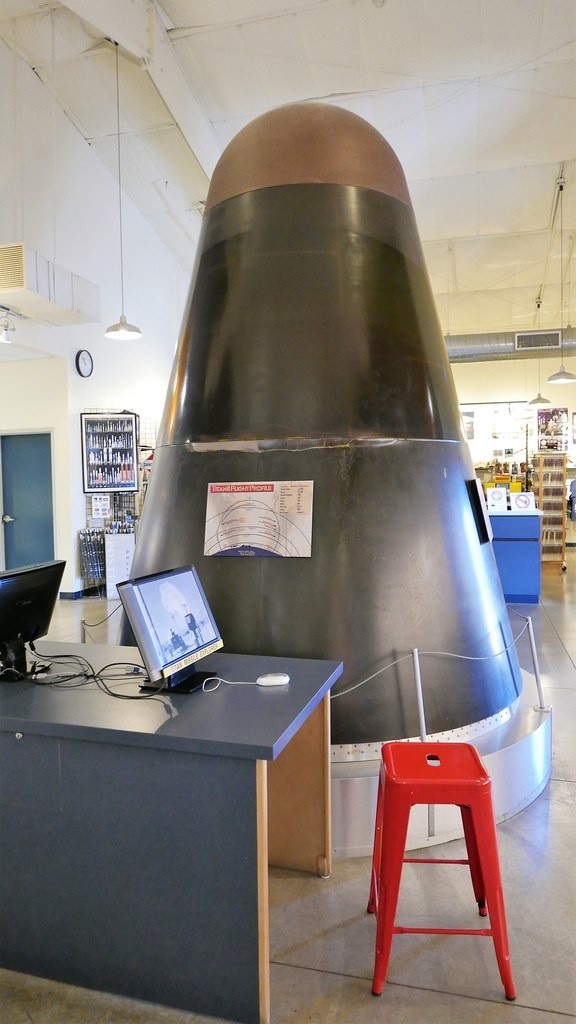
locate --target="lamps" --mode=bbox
[0,321,12,343]
[548,177,576,383]
[105,42,142,341]
[529,299,550,405]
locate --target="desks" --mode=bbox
[0,641,344,1024]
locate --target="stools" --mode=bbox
[366,743,516,1000]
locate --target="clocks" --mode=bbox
[75,350,94,377]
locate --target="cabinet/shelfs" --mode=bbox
[532,452,567,574]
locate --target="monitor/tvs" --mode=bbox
[0,559,66,681]
[116,563,223,693]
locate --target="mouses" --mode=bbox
[256,673,290,687]
